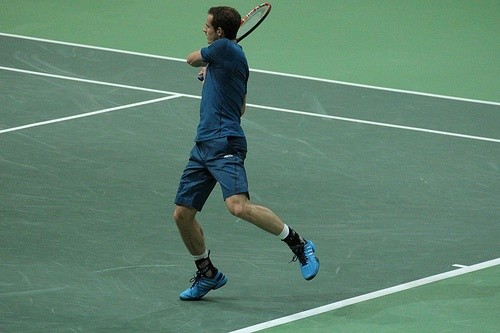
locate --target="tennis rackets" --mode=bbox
[198,1,272,83]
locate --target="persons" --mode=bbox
[172,4,319,301]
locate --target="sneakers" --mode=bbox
[288,238,320,281]
[179,268,227,300]
